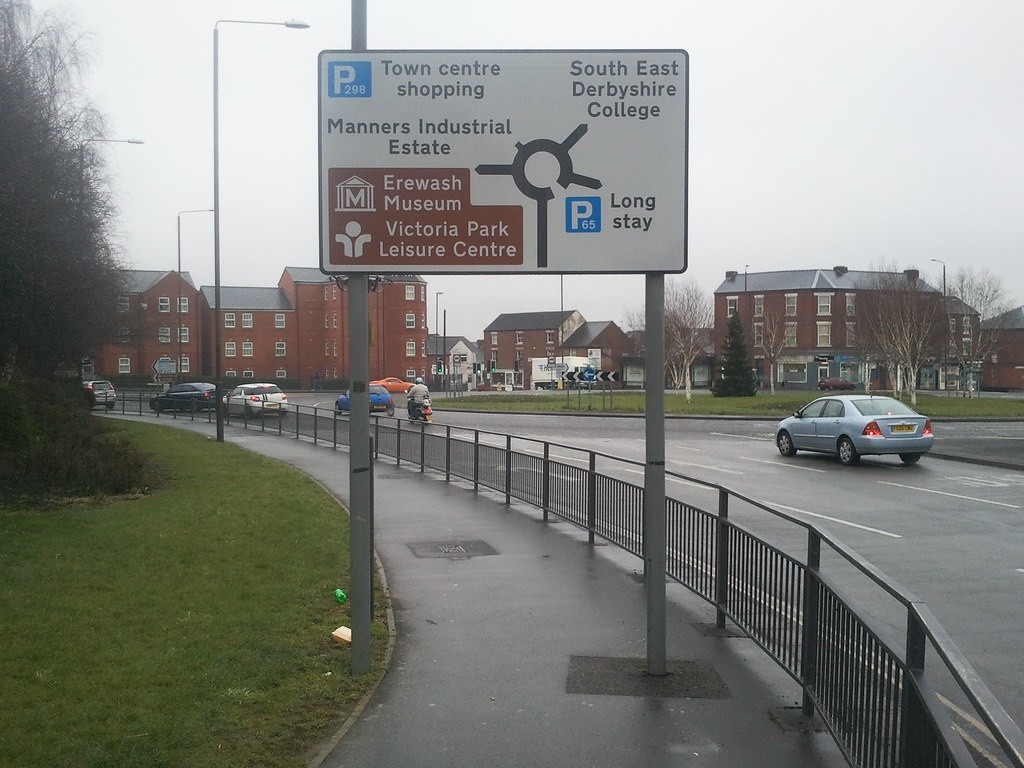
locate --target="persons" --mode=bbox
[407,376,429,419]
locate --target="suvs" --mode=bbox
[82,381,116,409]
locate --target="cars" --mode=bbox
[222,383,288,418]
[368,377,417,393]
[777,395,934,468]
[819,377,856,391]
[150,383,218,413]
[334,384,395,417]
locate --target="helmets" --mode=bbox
[416,377,424,384]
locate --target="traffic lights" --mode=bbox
[477,363,480,373]
[492,361,495,372]
[438,360,442,372]
[514,360,518,371]
[473,363,476,374]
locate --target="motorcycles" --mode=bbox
[405,389,433,424]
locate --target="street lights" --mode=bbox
[77,137,143,198]
[436,292,443,383]
[175,209,216,381]
[930,258,948,390]
[211,16,311,438]
